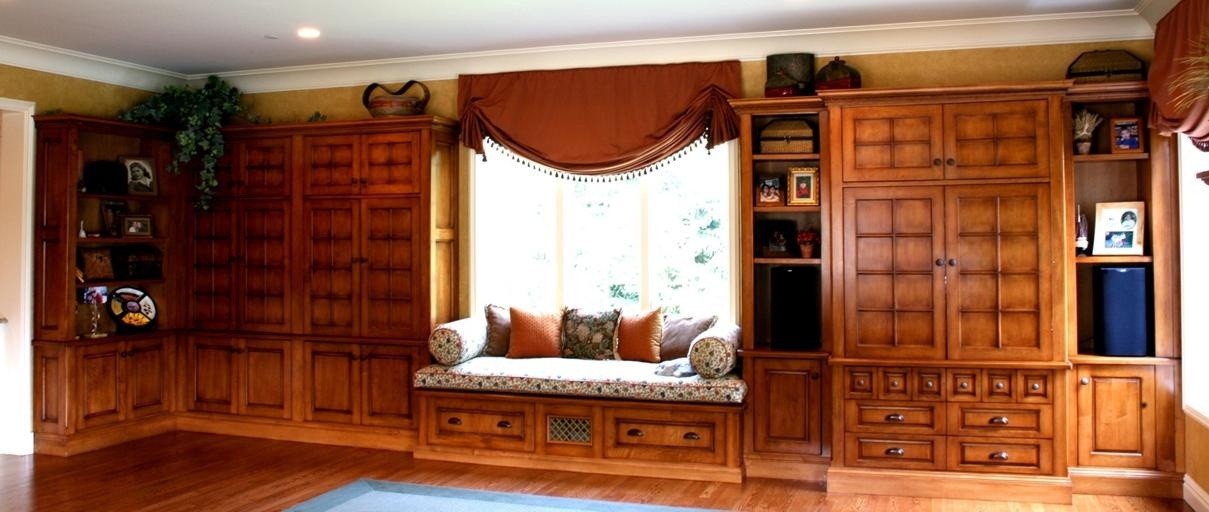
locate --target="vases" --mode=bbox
[1073,140,1094,155]
[799,238,817,259]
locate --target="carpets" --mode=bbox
[265,473,725,512]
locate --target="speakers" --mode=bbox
[1092,267,1145,358]
[772,265,821,352]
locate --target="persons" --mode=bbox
[1120,210,1137,231]
[797,179,809,197]
[768,185,780,202]
[136,221,146,232]
[129,221,138,233]
[1116,127,1139,149]
[125,161,150,191]
[759,184,770,202]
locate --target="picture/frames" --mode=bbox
[1085,197,1148,257]
[1106,113,1146,155]
[117,212,151,237]
[759,218,801,259]
[98,198,131,237]
[784,165,820,207]
[119,152,159,197]
[754,169,788,209]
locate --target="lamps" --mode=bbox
[73,283,113,339]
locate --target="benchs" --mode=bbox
[407,357,753,489]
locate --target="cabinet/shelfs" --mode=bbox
[949,363,1069,479]
[188,191,299,338]
[179,335,301,443]
[722,92,829,356]
[30,107,189,341]
[818,184,1082,369]
[37,341,179,458]
[194,124,303,192]
[300,336,421,455]
[298,197,461,341]
[303,113,460,198]
[601,397,736,472]
[814,71,1068,184]
[741,350,830,467]
[1064,81,1170,361]
[412,390,539,461]
[1069,356,1176,474]
[829,360,948,474]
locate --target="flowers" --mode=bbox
[795,223,820,246]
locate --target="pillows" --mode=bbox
[617,305,665,363]
[502,306,568,359]
[482,302,513,359]
[425,312,488,367]
[559,303,625,362]
[659,311,720,361]
[684,319,743,380]
